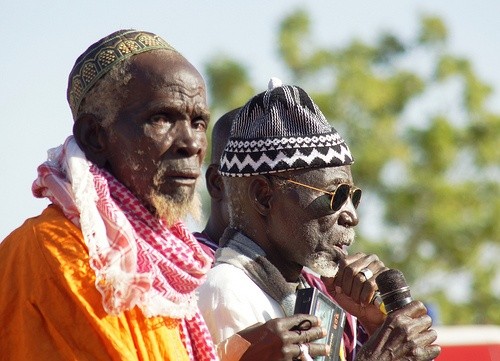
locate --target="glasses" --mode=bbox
[271,174,362,211]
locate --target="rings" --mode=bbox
[298,344,308,360]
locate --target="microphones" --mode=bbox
[377,268,414,314]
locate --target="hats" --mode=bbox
[66,29,176,124]
[217,85,355,178]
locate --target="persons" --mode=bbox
[192,105,242,263]
[0,29,331,361]
[204,87,440,361]
